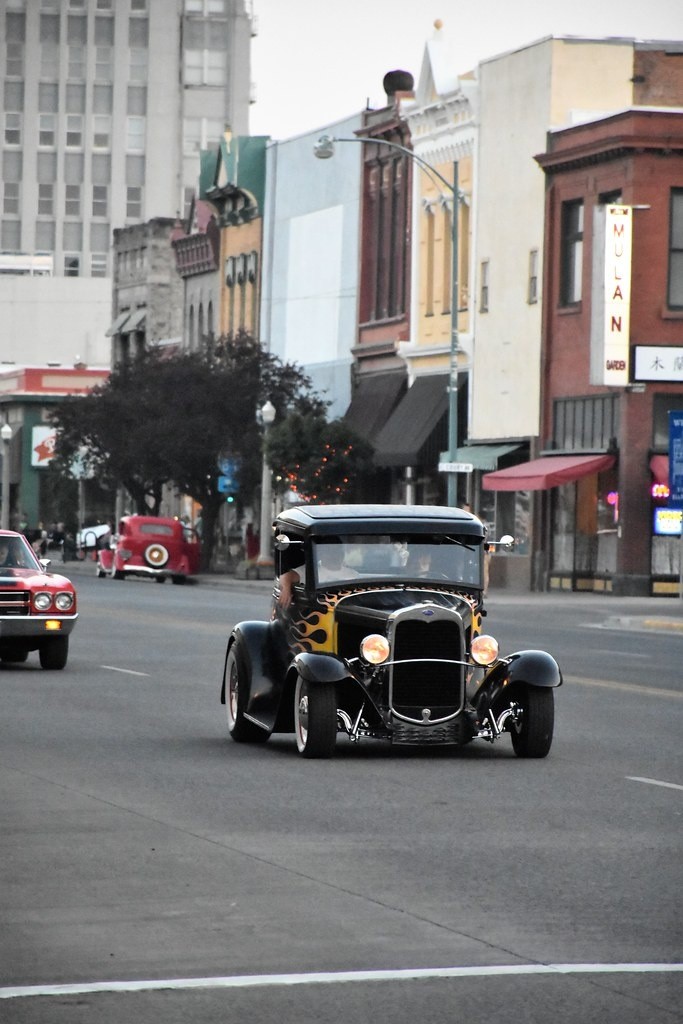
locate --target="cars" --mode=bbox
[97,516,201,585]
[219,504,563,760]
[0,529,78,672]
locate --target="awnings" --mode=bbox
[342,371,616,490]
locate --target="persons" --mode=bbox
[279,534,447,612]
[464,503,492,598]
[0,508,207,570]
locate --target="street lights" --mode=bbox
[313,133,459,507]
[0,422,13,530]
[257,400,277,580]
